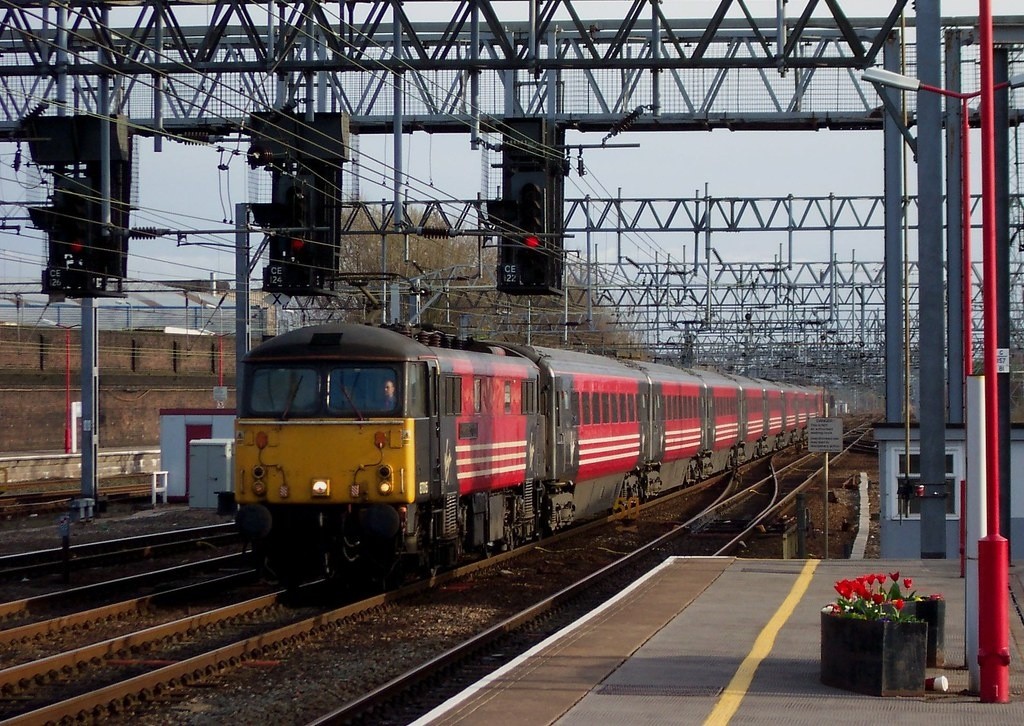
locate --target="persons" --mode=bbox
[372,380,397,410]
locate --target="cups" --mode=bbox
[925,676,948,691]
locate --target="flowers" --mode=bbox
[857,572,942,602]
[822,580,924,622]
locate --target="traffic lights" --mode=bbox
[50,177,98,293]
[274,174,317,269]
[508,173,553,286]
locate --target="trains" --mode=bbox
[227,318,832,606]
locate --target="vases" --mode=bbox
[820,612,929,698]
[890,600,945,668]
